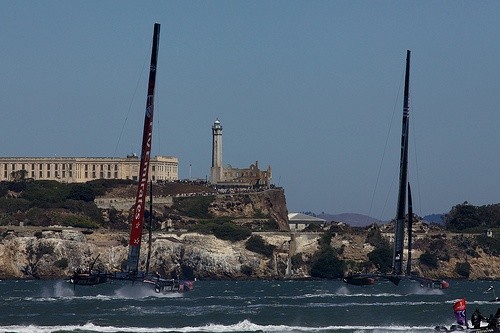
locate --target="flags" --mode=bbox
[452,299,466,327]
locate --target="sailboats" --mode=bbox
[342,45,449,290]
[67,20,195,297]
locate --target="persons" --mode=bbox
[68,266,163,284]
[346,263,397,280]
[471,308,483,329]
